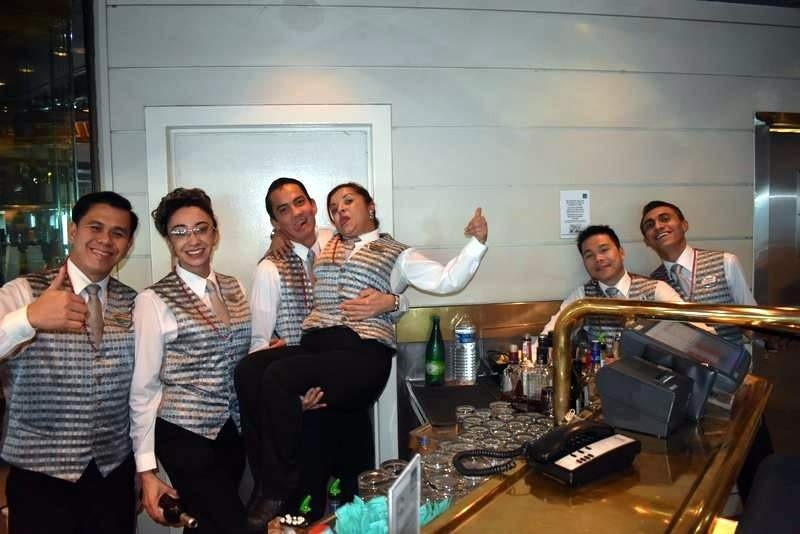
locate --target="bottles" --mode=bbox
[139,488,198,529]
[296,494,312,528]
[425,315,445,385]
[501,330,620,414]
[326,478,342,515]
[0,223,64,287]
[450,309,477,385]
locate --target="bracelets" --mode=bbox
[394,295,400,311]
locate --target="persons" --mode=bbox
[233,178,487,534]
[639,201,758,346]
[0,191,139,534]
[532,226,718,367]
[127,188,252,534]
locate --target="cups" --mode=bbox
[356,386,558,506]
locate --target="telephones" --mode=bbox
[526,420,642,488]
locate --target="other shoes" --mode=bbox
[248,498,285,526]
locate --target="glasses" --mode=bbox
[168,222,217,241]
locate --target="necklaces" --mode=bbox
[298,260,310,315]
[83,321,97,352]
[175,268,228,342]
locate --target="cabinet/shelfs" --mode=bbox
[0,4,79,287]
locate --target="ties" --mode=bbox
[206,279,231,326]
[605,287,619,298]
[306,249,316,285]
[670,264,691,297]
[85,285,104,347]
[343,235,360,260]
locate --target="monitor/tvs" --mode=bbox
[636,322,753,393]
